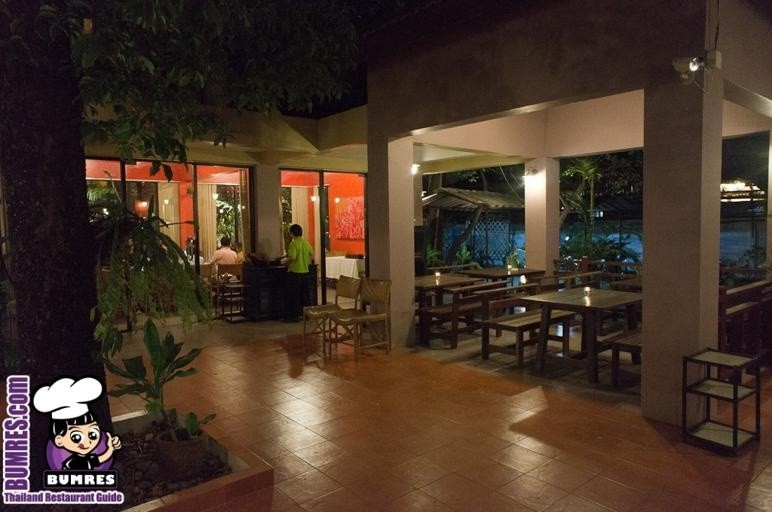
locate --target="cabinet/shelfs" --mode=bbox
[682,347,761,457]
[222,283,252,324]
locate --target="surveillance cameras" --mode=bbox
[671,56,699,73]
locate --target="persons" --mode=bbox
[207,237,236,272]
[280,224,315,320]
[234,242,243,263]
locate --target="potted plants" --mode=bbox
[98,318,216,473]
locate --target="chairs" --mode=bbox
[215,263,245,319]
[303,275,363,360]
[190,263,213,301]
[329,278,392,363]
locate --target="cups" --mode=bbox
[584,286,592,294]
[435,271,441,281]
[506,264,513,272]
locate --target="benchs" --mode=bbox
[415,265,643,387]
